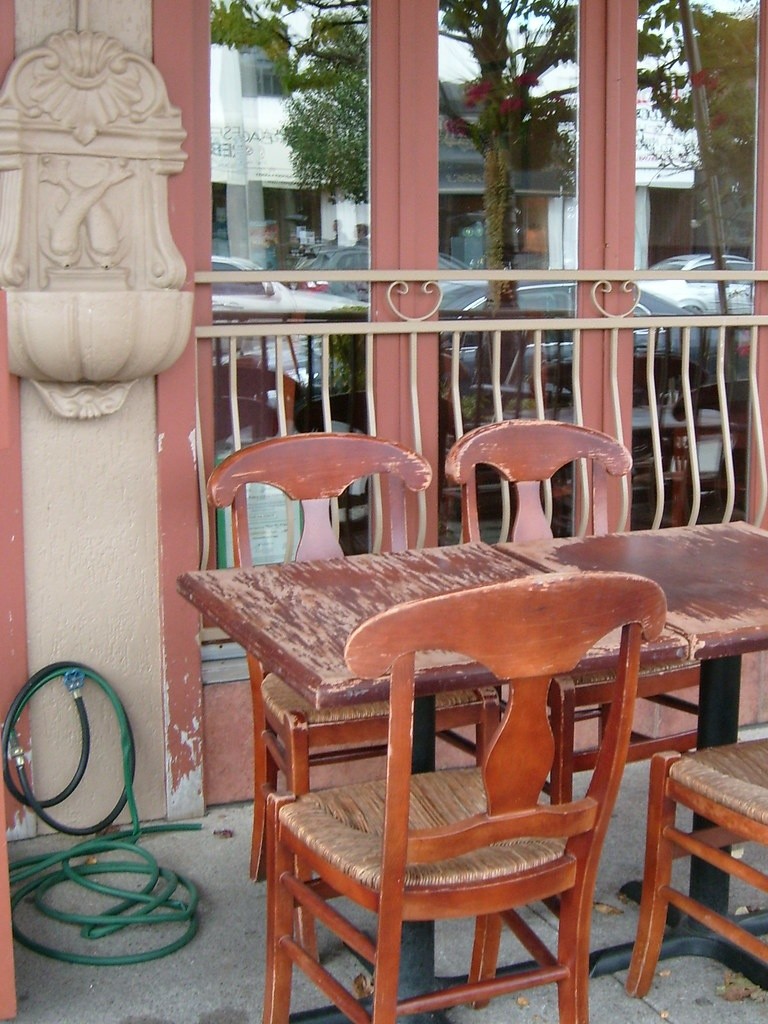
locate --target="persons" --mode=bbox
[327,219,338,245]
[353,223,369,246]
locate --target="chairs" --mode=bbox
[670,380,752,529]
[204,432,497,968]
[625,737,768,1004]
[442,417,704,912]
[263,571,664,1024]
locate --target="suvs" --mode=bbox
[289,245,474,301]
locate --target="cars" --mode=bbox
[211,254,362,354]
[632,254,755,328]
[436,268,754,415]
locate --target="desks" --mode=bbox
[178,540,687,1024]
[490,520,768,1001]
[494,404,736,530]
